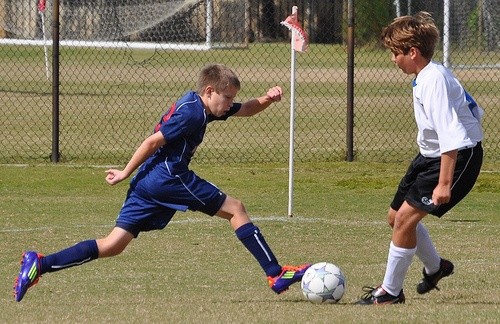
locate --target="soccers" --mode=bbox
[300,262,346,304]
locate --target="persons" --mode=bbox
[355,13,484,305]
[13,63,316,304]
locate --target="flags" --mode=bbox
[281,12,308,54]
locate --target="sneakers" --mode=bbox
[14,250,45,302]
[417,257,454,294]
[354,287,406,306]
[267,264,311,294]
